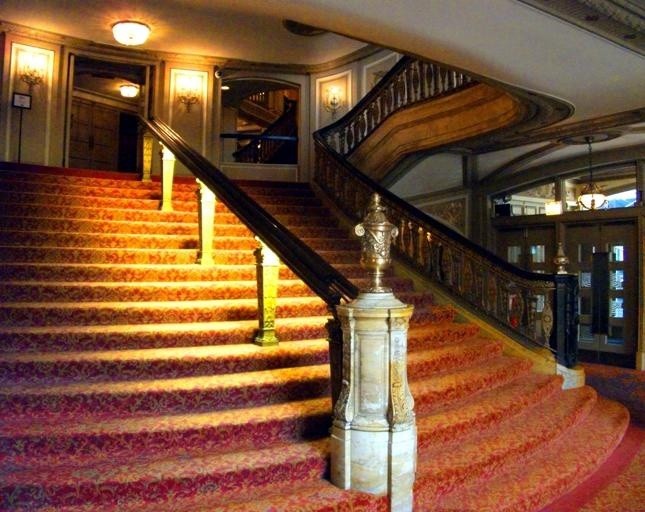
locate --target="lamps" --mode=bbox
[324,87,343,119]
[119,84,140,99]
[577,136,608,212]
[19,58,44,95]
[110,21,151,45]
[177,83,200,113]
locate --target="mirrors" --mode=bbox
[220,77,301,165]
